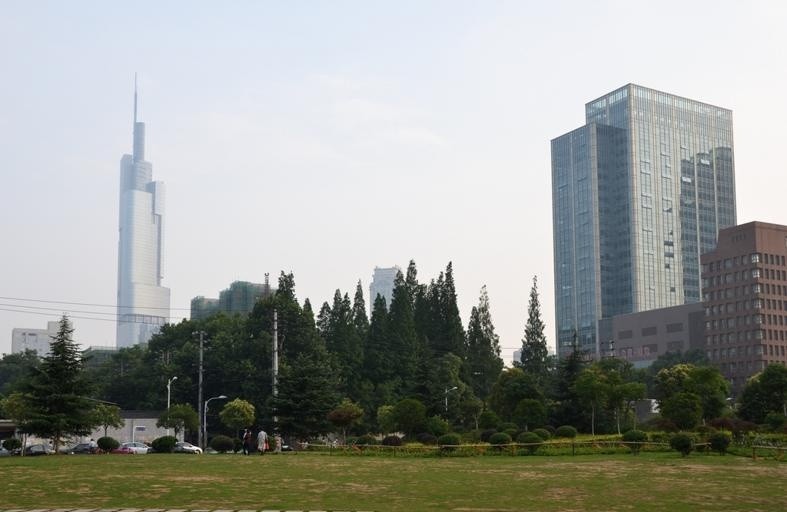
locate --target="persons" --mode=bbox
[273,434,284,454]
[243,429,250,455]
[242,428,251,454]
[47,441,52,455]
[88,438,95,454]
[256,429,268,456]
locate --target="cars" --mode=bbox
[0,441,154,456]
[173,442,202,455]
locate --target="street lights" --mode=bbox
[203,394,227,449]
[444,387,460,424]
[166,376,179,435]
[191,330,207,454]
